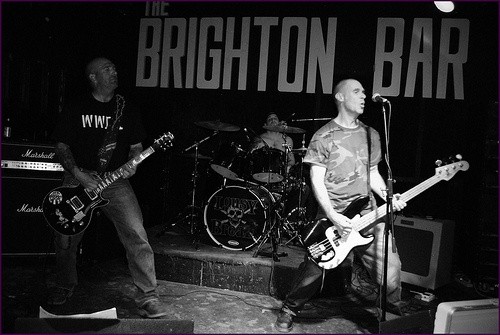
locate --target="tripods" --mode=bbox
[253,129,311,263]
[160,131,218,237]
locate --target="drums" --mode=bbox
[272,176,309,225]
[208,138,251,180]
[200,181,278,252]
[248,146,289,184]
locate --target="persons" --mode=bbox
[274,79,407,333]
[250,112,296,174]
[46,57,167,319]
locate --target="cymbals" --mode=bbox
[200,120,241,132]
[290,146,309,154]
[260,124,308,134]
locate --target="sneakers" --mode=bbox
[376,293,409,315]
[275,304,296,332]
[133,290,168,317]
[45,282,74,304]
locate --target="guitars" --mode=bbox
[296,152,472,272]
[40,132,174,237]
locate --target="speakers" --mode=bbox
[12,317,195,334]
[2,169,82,259]
[387,214,453,289]
[431,297,498,334]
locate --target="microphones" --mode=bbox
[371,93,391,104]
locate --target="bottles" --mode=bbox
[4,116,12,139]
